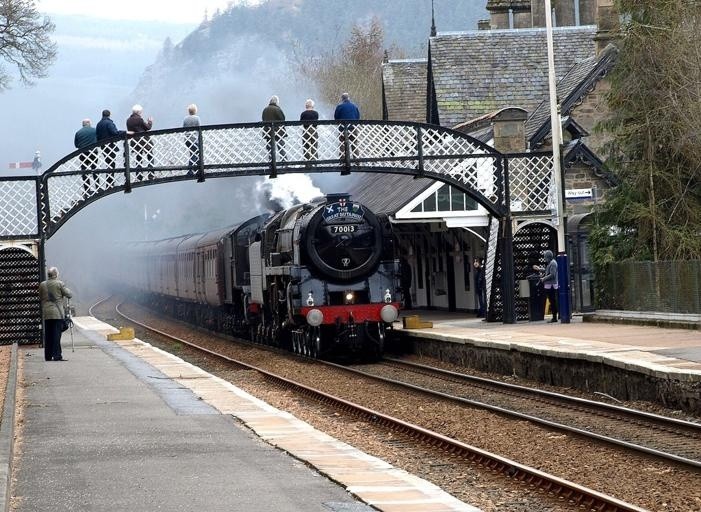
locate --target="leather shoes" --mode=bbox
[61,359,68,361]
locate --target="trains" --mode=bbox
[108,190,405,362]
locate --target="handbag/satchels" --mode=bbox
[61,316,70,331]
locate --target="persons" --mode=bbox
[261,95,290,169]
[182,103,201,175]
[74,120,103,198]
[126,104,154,181]
[39,267,73,361]
[533,249,559,323]
[472,258,481,317]
[396,256,414,310]
[95,109,136,191]
[334,93,361,166]
[300,99,318,168]
[477,260,487,321]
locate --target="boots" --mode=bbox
[547,313,557,323]
